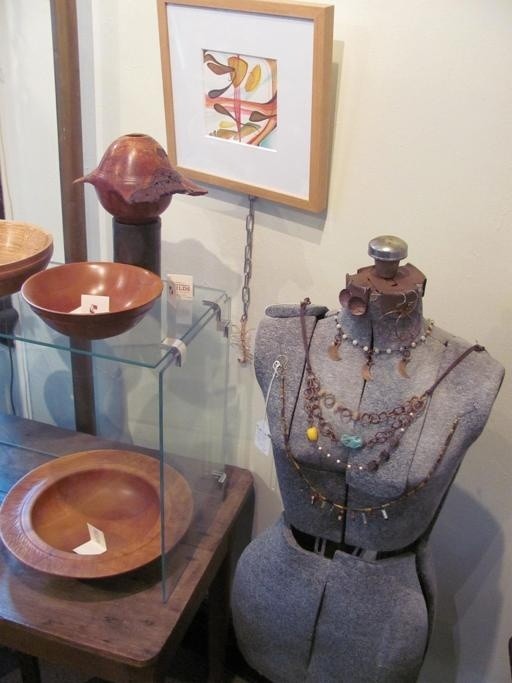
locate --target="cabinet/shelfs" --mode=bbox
[1,258,256,681]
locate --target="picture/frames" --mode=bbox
[157,0,334,214]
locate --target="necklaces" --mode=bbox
[269,298,487,527]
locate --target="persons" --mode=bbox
[230,261,504,681]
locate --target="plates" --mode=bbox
[0,446,196,583]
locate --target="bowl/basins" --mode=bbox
[0,217,56,301]
[19,258,167,341]
